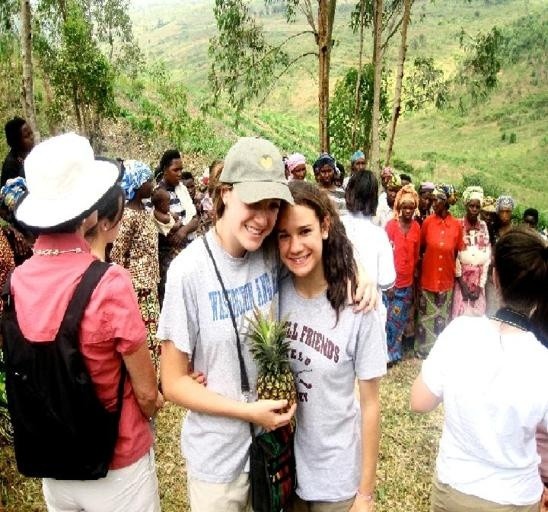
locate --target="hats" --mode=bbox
[13,132,121,232]
[219,135,296,207]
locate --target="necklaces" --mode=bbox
[30,246,85,257]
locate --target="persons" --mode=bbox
[1,132,164,511]
[188,180,391,512]
[409,222,546,512]
[154,134,383,512]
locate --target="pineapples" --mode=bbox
[257,367,297,413]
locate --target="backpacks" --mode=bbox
[0,258,127,481]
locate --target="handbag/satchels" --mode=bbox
[248,423,298,512]
